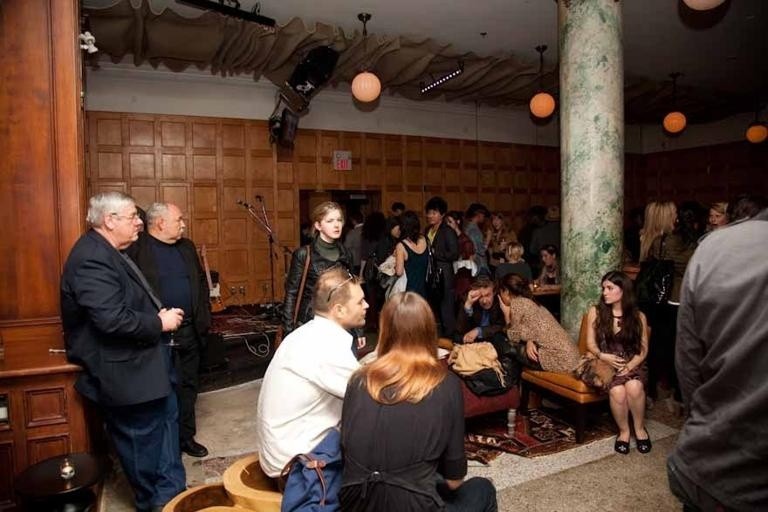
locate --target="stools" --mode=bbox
[160,448,282,511]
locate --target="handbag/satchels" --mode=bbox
[448,341,498,377]
[634,261,674,306]
[281,427,343,512]
[363,251,389,293]
[464,368,512,396]
[426,256,442,288]
[576,351,616,388]
[274,319,302,352]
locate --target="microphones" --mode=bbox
[237,200,255,209]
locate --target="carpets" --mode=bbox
[461,405,625,468]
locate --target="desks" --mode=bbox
[9,450,117,510]
[530,279,559,300]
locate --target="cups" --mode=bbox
[614,351,626,372]
[60,461,74,479]
[534,280,540,288]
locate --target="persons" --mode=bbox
[126,201,212,458]
[59,189,187,512]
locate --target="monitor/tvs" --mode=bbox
[271,108,299,149]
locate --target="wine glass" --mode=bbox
[163,307,181,347]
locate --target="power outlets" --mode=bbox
[262,284,270,292]
[227,285,236,293]
[239,287,244,295]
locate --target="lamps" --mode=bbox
[171,0,277,33]
[417,61,464,95]
[661,72,687,135]
[529,44,556,119]
[352,13,382,104]
[681,0,726,11]
[746,86,767,143]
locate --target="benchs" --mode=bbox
[521,308,651,444]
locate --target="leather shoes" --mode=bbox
[180,438,208,457]
[633,426,650,453]
[615,432,629,454]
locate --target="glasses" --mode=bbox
[322,261,355,302]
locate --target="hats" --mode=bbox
[470,204,490,217]
[545,205,561,221]
